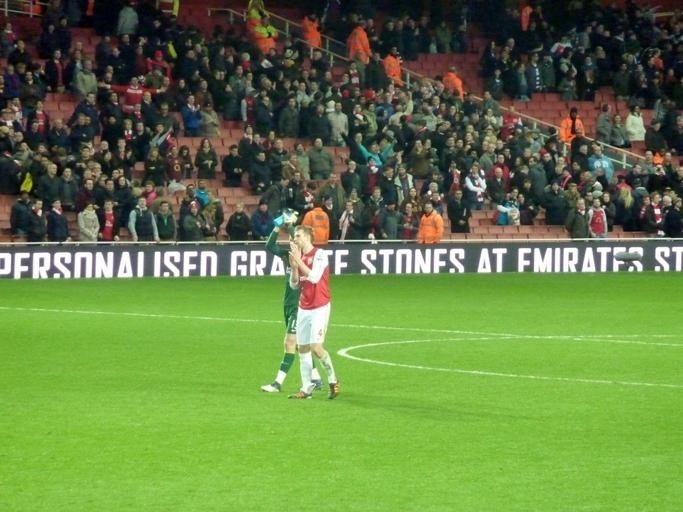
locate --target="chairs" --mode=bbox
[2,4,664,238]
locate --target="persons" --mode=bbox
[286,224,342,400]
[0,0,683,245]
[259,205,325,392]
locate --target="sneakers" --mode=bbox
[286,392,312,400]
[310,378,322,390]
[327,379,340,401]
[260,381,281,393]
